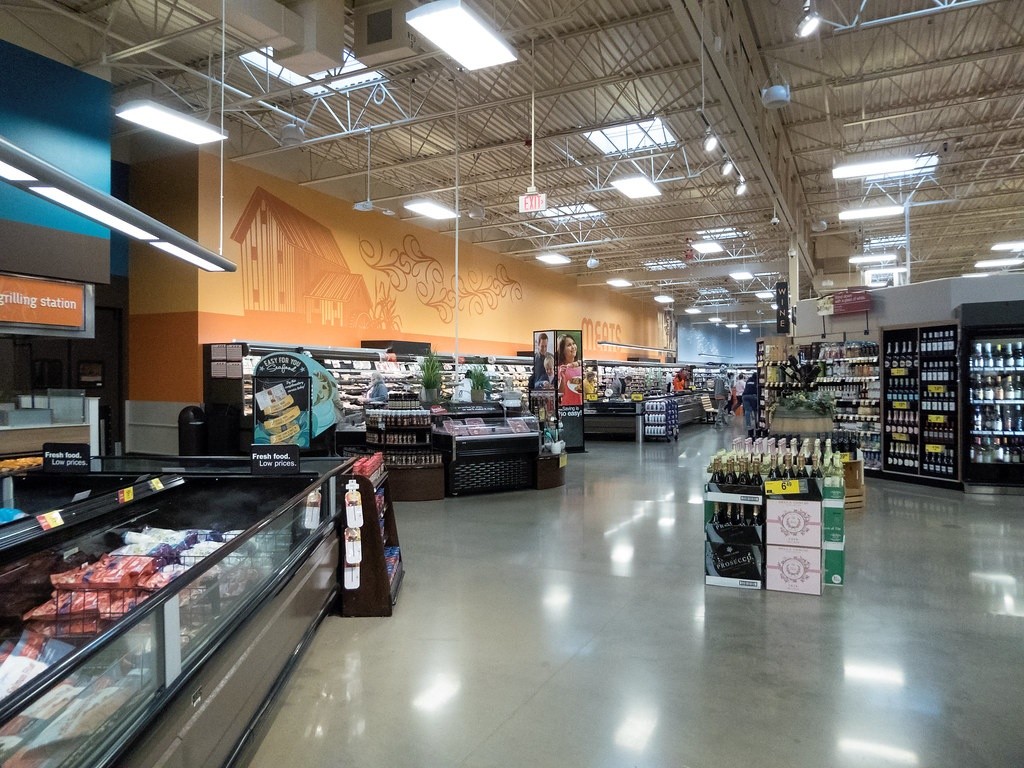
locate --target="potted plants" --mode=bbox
[471,368,492,403]
[417,350,442,403]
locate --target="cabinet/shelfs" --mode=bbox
[754,301,1024,490]
[334,458,405,617]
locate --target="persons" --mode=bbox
[457,370,473,402]
[663,372,673,393]
[343,372,388,422]
[742,372,762,427]
[534,333,554,390]
[557,334,582,406]
[583,372,597,401]
[610,376,632,399]
[713,370,731,429]
[727,372,746,416]
[674,368,691,391]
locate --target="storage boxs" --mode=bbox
[704,493,845,595]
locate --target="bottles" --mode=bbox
[885,329,957,480]
[757,342,767,429]
[345,394,442,464]
[818,341,881,468]
[968,341,1024,466]
[708,436,843,526]
[780,355,820,385]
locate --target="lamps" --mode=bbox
[0,0,1023,332]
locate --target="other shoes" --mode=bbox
[730,410,736,416]
